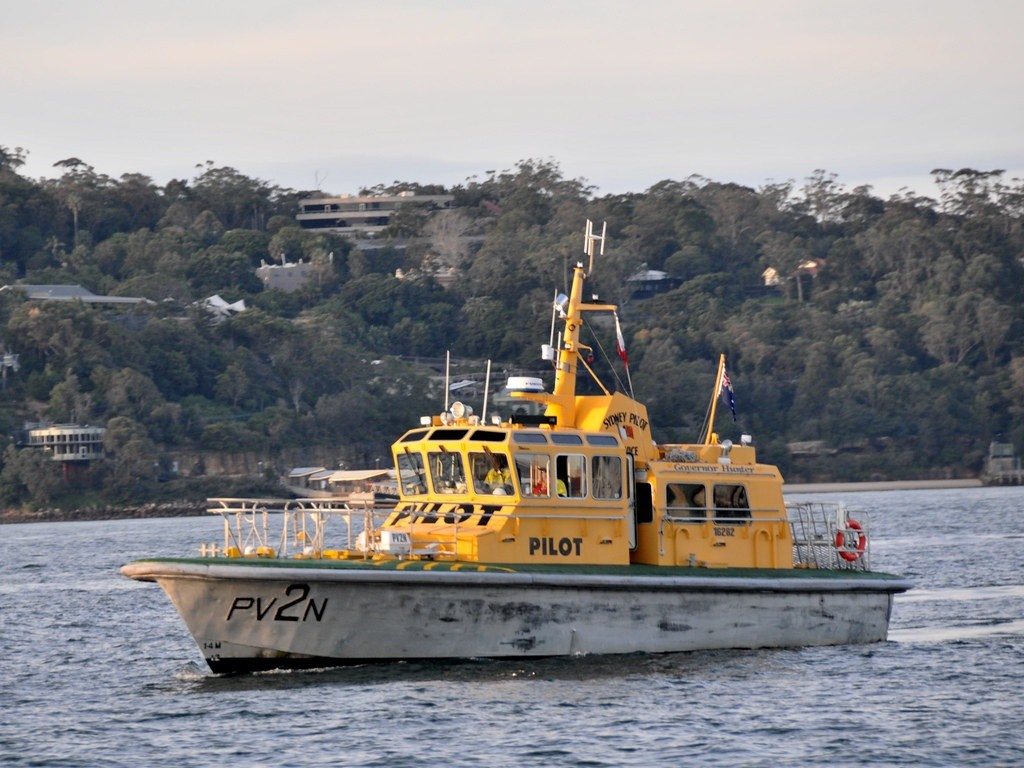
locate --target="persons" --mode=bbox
[533,465,567,498]
[481,452,515,496]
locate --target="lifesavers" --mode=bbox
[833,518,870,563]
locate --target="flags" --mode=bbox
[715,362,737,422]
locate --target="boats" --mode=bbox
[113,223,916,680]
